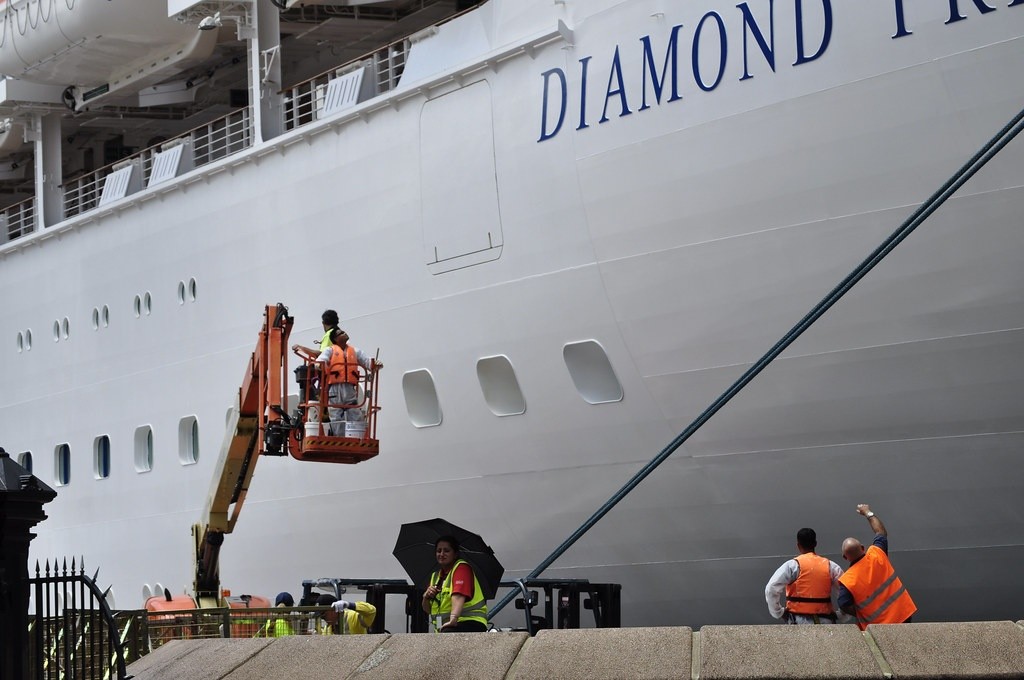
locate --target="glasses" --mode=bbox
[336,331,345,337]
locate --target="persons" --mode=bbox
[836,504,917,626]
[422,538,487,632]
[314,328,382,435]
[765,528,852,623]
[309,594,376,634]
[320,310,339,351]
[265,592,297,637]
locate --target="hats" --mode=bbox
[276,592,294,606]
[312,594,338,619]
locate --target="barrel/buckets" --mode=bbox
[307,401,320,422]
[305,421,330,437]
[345,421,365,439]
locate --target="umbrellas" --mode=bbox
[392,517,504,599]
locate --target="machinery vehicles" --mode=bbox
[143,304,381,646]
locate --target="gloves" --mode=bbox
[331,600,349,613]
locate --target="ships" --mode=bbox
[0,0,1023,680]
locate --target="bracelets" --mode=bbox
[450,614,456,618]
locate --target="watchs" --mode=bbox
[867,512,875,519]
